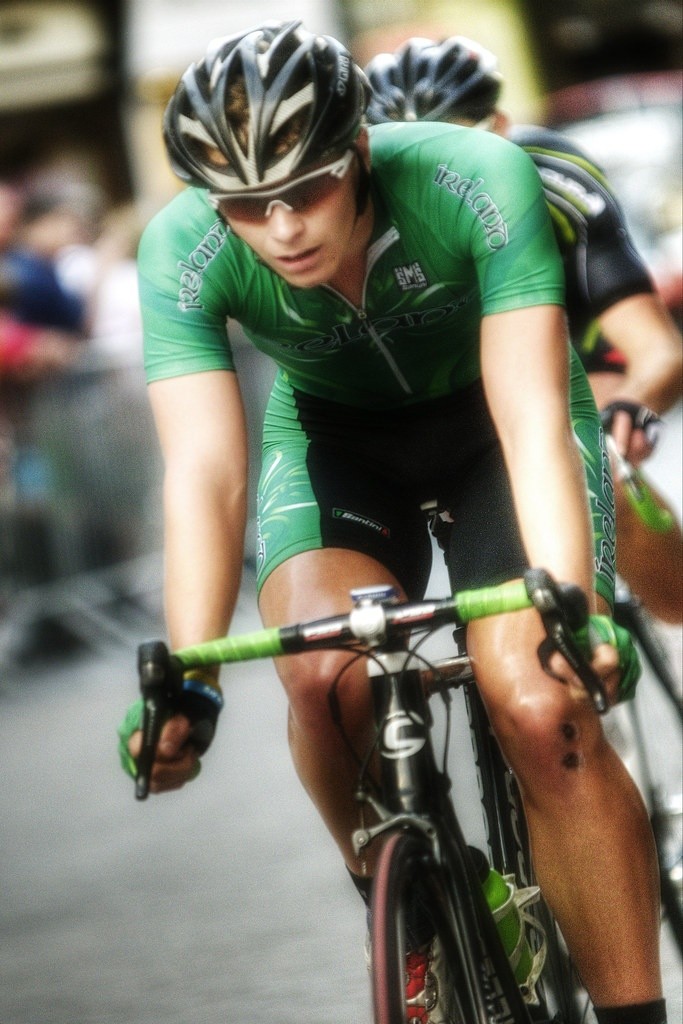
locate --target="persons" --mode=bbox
[119,22,668,1024]
[359,35,683,627]
[0,189,95,376]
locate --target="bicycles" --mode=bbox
[131,497,682,1024]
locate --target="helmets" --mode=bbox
[157,20,372,203]
[368,35,501,125]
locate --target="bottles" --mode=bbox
[466,844,534,985]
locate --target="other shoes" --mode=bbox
[361,929,465,1023]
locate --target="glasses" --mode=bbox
[210,149,356,223]
